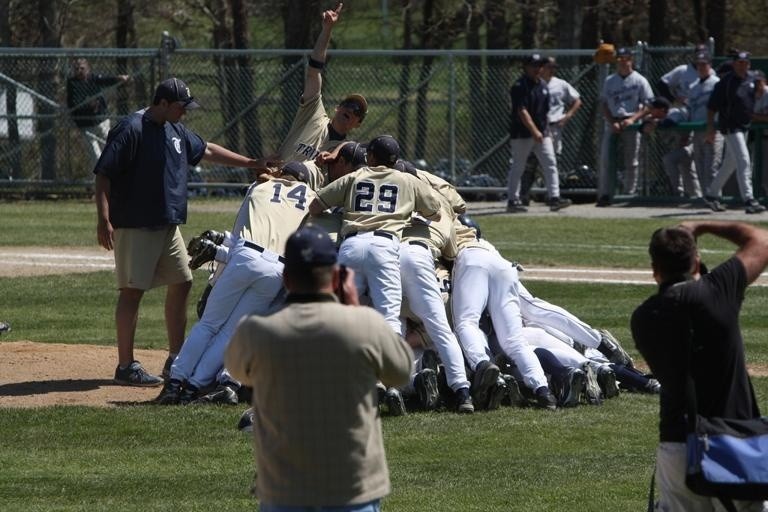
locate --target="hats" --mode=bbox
[616,48,633,57]
[347,93,368,115]
[523,53,560,69]
[646,96,669,113]
[285,227,338,264]
[339,135,416,176]
[458,214,481,239]
[693,45,766,79]
[156,78,201,110]
[282,162,309,184]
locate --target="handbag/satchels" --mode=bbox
[684,414,768,501]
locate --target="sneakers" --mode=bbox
[376,329,662,416]
[154,357,253,405]
[187,230,225,270]
[746,199,766,213]
[115,361,165,387]
[507,195,529,213]
[702,195,727,211]
[547,197,572,211]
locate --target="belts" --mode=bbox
[244,241,285,264]
[409,241,428,249]
[345,232,392,239]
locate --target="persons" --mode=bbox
[92,76,279,388]
[639,46,768,215]
[154,1,660,432]
[224,224,415,512]
[65,59,129,163]
[631,219,768,512]
